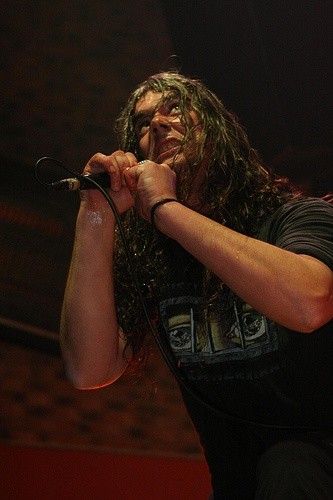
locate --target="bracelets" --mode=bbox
[149,197,180,229]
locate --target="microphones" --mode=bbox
[50,171,111,192]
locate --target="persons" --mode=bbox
[59,71,333,500]
